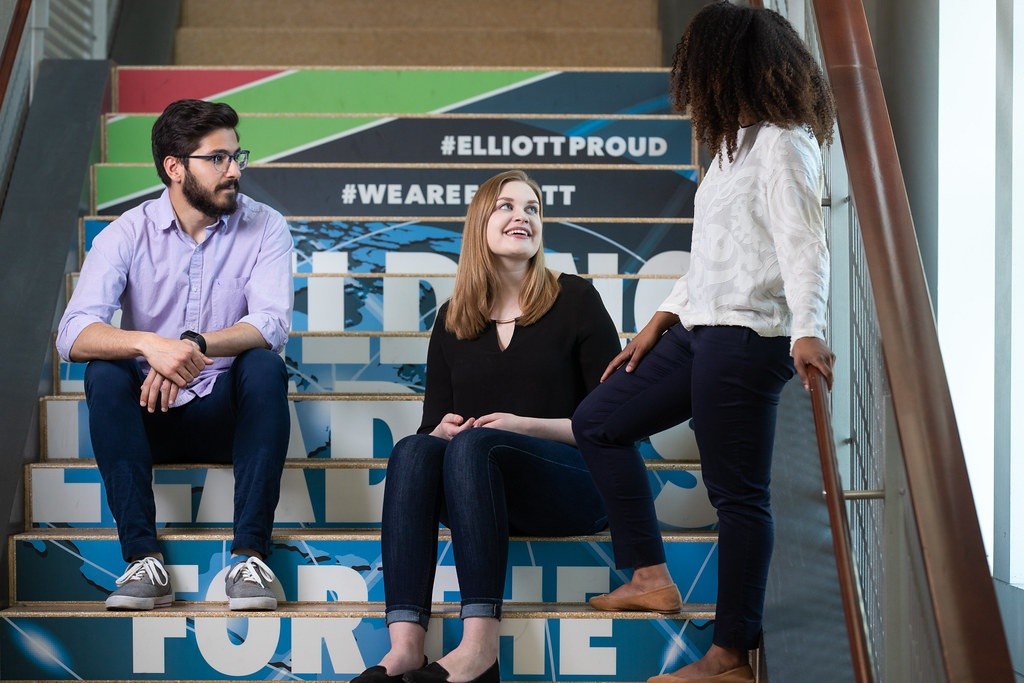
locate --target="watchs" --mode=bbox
[179,329,208,354]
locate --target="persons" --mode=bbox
[350,168,628,683]
[569,7,837,681]
[55,100,294,611]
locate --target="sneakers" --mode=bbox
[225,561,277,611]
[104,558,172,611]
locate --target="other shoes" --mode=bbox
[589,583,683,613]
[648,664,756,683]
[350,655,428,683]
[402,659,502,683]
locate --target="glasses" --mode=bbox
[174,149,250,173]
